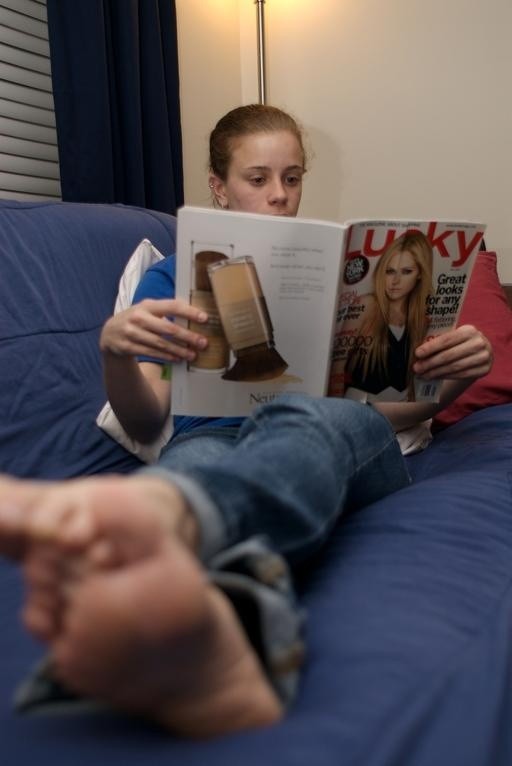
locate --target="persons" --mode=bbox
[331,229,434,402]
[0,104,495,740]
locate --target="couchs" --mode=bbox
[1,199,512,766]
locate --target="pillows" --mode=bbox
[430,249,512,423]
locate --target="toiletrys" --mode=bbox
[204,255,288,381]
[187,251,232,374]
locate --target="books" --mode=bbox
[168,207,488,420]
